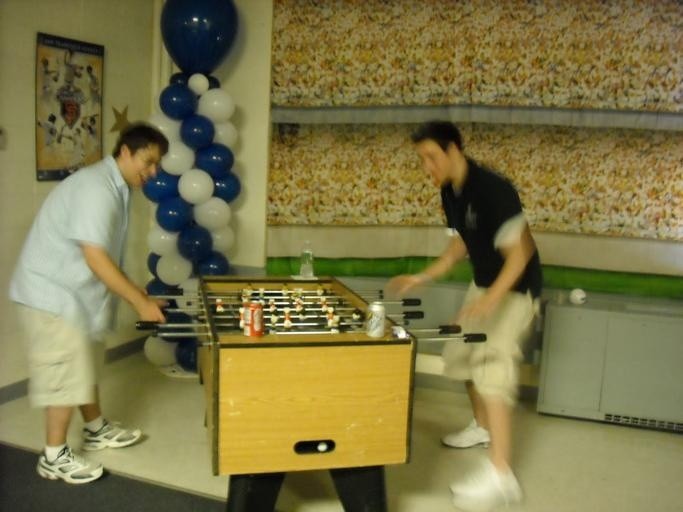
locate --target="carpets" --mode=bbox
[0,434,232,510]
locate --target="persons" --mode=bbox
[6,123,171,487]
[386,121,547,506]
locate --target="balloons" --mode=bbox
[139,68,242,373]
[160,0,237,74]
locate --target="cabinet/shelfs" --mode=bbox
[528,291,682,432]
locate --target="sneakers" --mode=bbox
[79,416,143,453]
[439,416,492,449]
[34,444,108,486]
[447,456,524,512]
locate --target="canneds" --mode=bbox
[244,302,264,337]
[366,301,385,338]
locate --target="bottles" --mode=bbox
[299,237,314,278]
